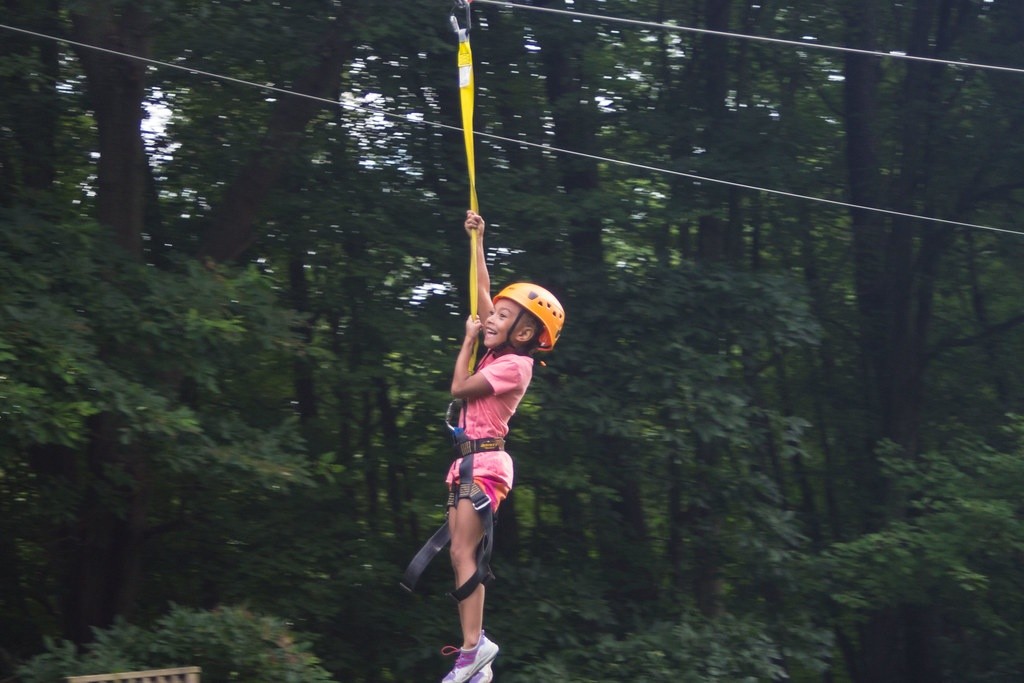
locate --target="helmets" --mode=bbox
[493,283,566,351]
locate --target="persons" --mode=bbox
[439,209,565,683]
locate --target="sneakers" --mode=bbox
[442,630,499,683]
[469,654,495,683]
